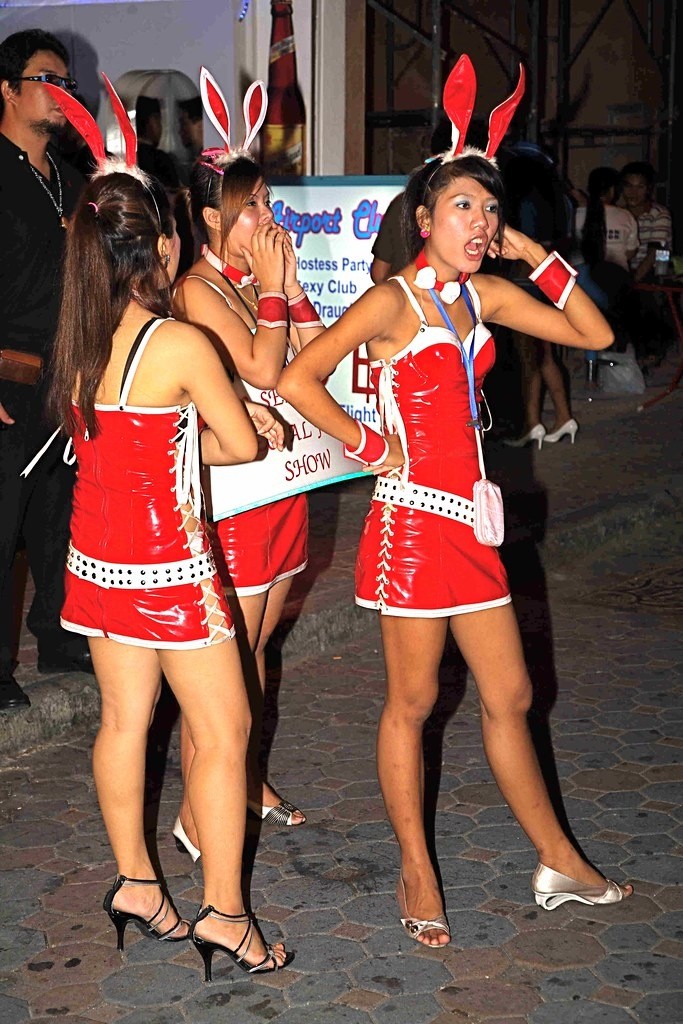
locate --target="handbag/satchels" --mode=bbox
[597,344,645,395]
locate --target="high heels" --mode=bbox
[102,873,193,951]
[187,904,295,982]
[531,862,631,910]
[504,422,546,451]
[543,418,579,445]
[396,868,450,949]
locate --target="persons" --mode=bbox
[275,154,633,948]
[172,157,337,866]
[40,171,284,982]
[136,95,204,186]
[0,29,95,710]
[430,115,671,451]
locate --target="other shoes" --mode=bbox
[247,792,307,827]
[172,815,205,871]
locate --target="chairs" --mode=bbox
[568,262,633,401]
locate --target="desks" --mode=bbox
[632,277,683,412]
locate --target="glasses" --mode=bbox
[17,74,79,92]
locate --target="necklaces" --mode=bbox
[30,152,69,229]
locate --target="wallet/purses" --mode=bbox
[472,478,505,546]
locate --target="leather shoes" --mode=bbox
[36,644,94,676]
[0,677,31,710]
